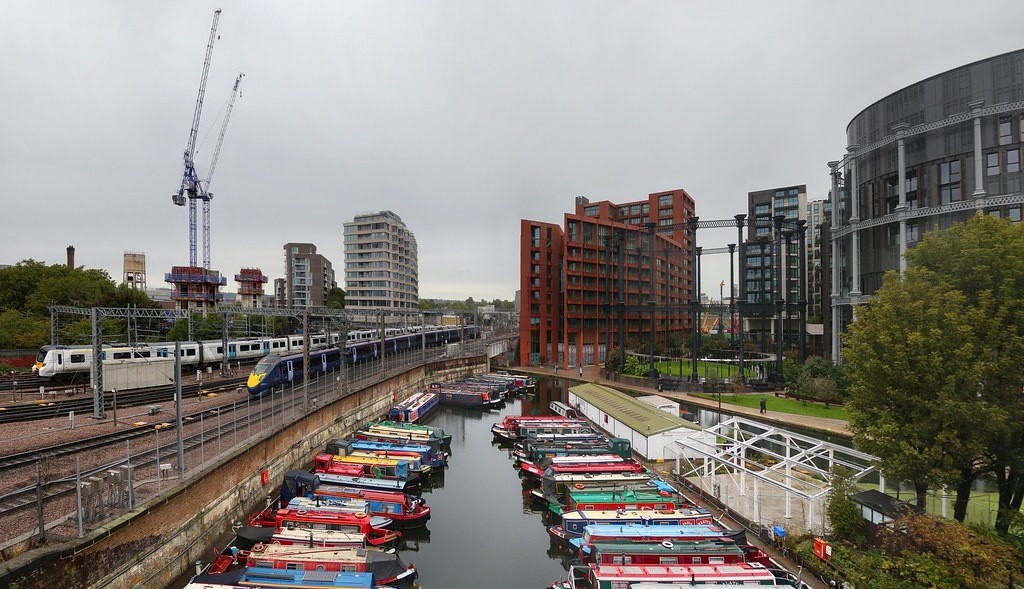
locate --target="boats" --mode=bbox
[492,401,807,589]
[181,422,453,589]
[387,371,538,424]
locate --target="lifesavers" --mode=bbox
[662,541,674,549]
[297,509,308,514]
[562,423,569,427]
[593,439,599,443]
[565,444,572,449]
[253,544,265,552]
[374,424,379,427]
[354,511,367,518]
[658,490,669,496]
[616,508,628,515]
[574,483,585,489]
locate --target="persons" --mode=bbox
[829,580,837,589]
[760,398,767,413]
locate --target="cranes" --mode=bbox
[171,9,247,276]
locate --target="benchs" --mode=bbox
[775,392,789,398]
[798,396,843,404]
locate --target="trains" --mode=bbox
[246,326,481,396]
[34,322,477,383]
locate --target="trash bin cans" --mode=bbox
[658,384,663,392]
[605,372,615,381]
